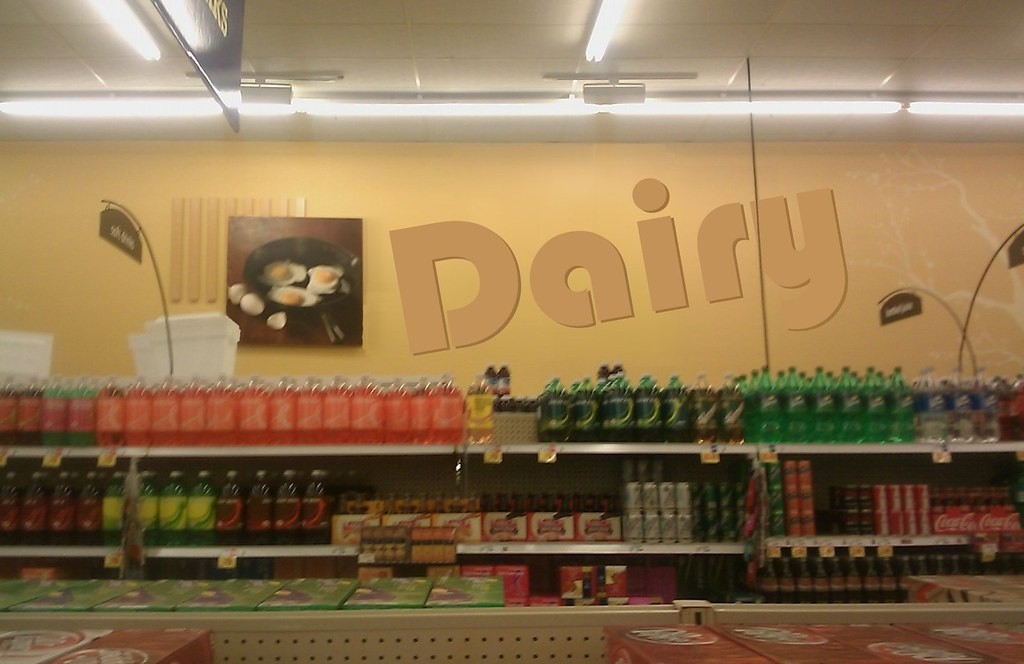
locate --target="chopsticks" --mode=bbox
[321,313,345,344]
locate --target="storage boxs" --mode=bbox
[0,513,678,664]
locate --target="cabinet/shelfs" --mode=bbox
[0,439,1024,581]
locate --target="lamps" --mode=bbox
[0,0,1024,116]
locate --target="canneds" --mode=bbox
[827,483,933,535]
[751,459,814,536]
[621,480,747,544]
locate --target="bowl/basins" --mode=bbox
[244,236,362,313]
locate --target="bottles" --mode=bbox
[898,487,1024,603]
[485,364,497,397]
[98,374,463,447]
[747,556,829,603]
[862,555,897,603]
[480,493,526,513]
[0,375,97,446]
[537,362,717,444]
[386,492,432,513]
[433,491,479,512]
[830,557,861,603]
[335,492,384,514]
[465,371,492,445]
[718,365,914,444]
[359,527,457,564]
[530,492,575,512]
[576,492,620,512]
[497,364,510,397]
[0,470,330,544]
[914,366,1024,444]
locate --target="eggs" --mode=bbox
[306,263,342,295]
[267,285,322,306]
[256,261,307,286]
